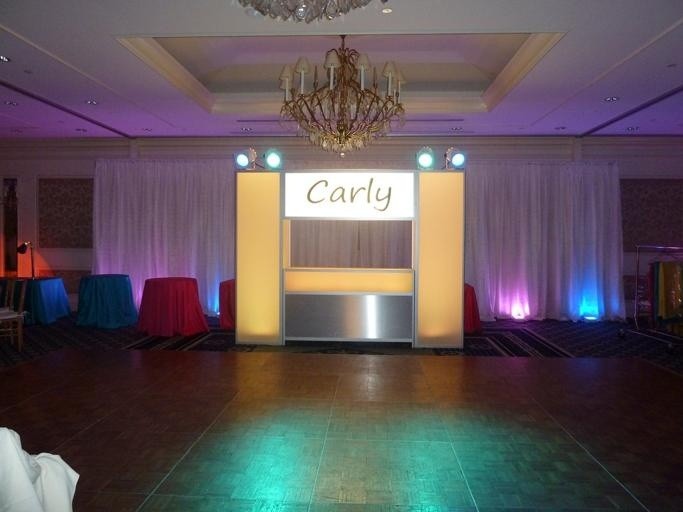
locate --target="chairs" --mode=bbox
[0,277,26,353]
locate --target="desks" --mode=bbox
[24,273,236,338]
[463,282,482,336]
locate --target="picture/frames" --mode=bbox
[36,176,94,251]
[619,176,683,252]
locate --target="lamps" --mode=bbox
[16,241,36,281]
[236,1,393,25]
[235,147,283,171]
[276,34,408,158]
[415,145,467,170]
